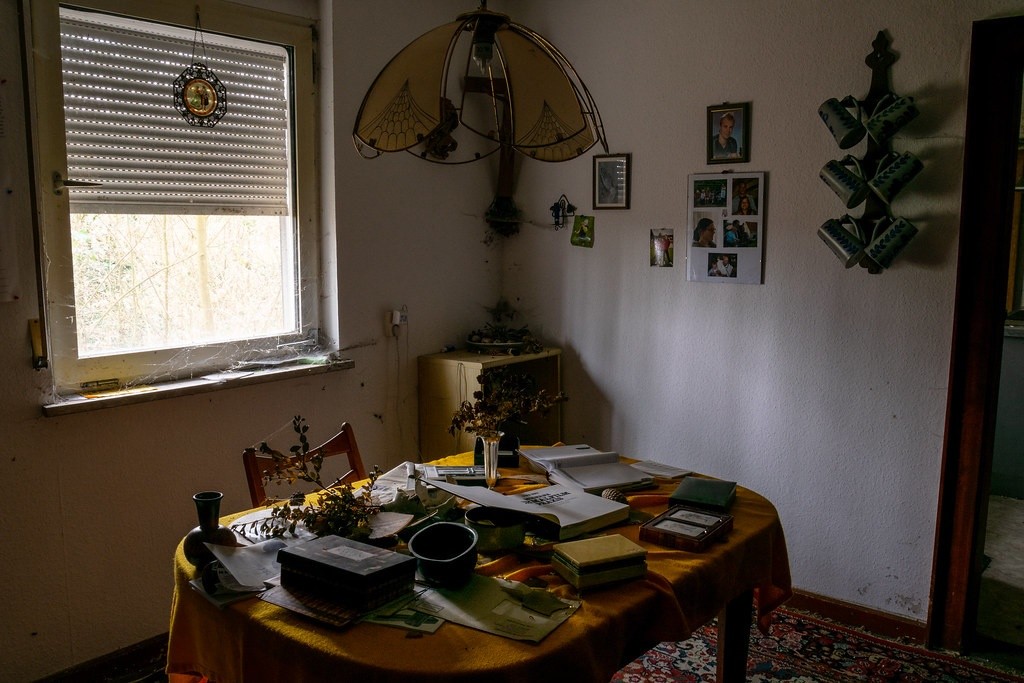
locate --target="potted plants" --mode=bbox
[445,363,570,490]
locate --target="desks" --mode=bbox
[173,444,777,683]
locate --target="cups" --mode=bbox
[408,521,478,587]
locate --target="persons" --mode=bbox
[733,197,758,215]
[695,184,726,205]
[708,256,734,277]
[713,112,737,157]
[576,218,589,238]
[692,218,717,248]
[661,234,672,264]
[726,220,753,247]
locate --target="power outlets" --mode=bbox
[383,310,404,338]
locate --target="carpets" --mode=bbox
[606,601,1024,683]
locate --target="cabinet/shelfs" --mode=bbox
[417,347,562,464]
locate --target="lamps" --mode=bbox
[350,0,610,165]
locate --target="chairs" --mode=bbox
[242,420,368,509]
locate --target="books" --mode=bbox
[516,444,655,495]
[418,477,629,539]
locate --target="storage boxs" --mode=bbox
[639,504,735,554]
[668,476,737,515]
[277,533,417,613]
[551,534,648,599]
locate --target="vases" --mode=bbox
[183,490,238,577]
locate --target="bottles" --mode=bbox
[184,491,236,572]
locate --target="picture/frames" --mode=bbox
[707,102,750,164]
[594,153,631,213]
